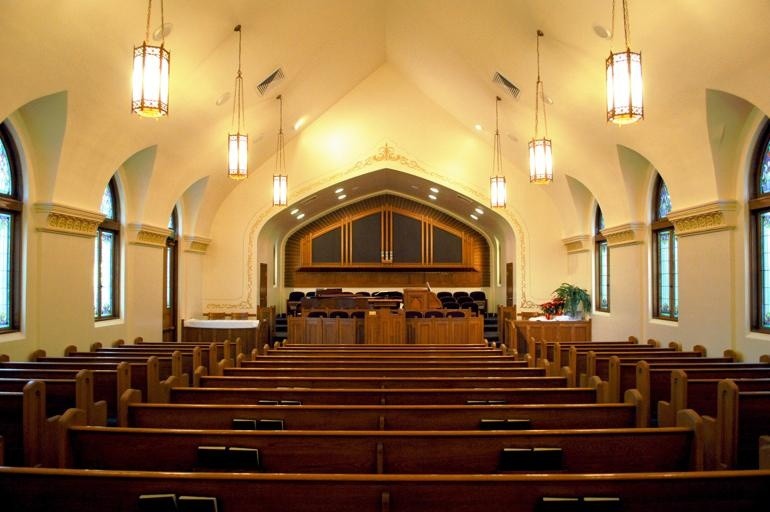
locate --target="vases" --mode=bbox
[544,310,565,321]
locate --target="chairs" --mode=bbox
[283,288,489,324]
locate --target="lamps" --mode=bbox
[489,99,507,209]
[128,1,171,116]
[526,32,555,184]
[225,28,248,178]
[603,1,645,125]
[273,99,287,206]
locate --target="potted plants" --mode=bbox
[556,281,592,318]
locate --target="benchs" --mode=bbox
[0,334,547,335]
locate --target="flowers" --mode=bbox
[541,298,565,313]
